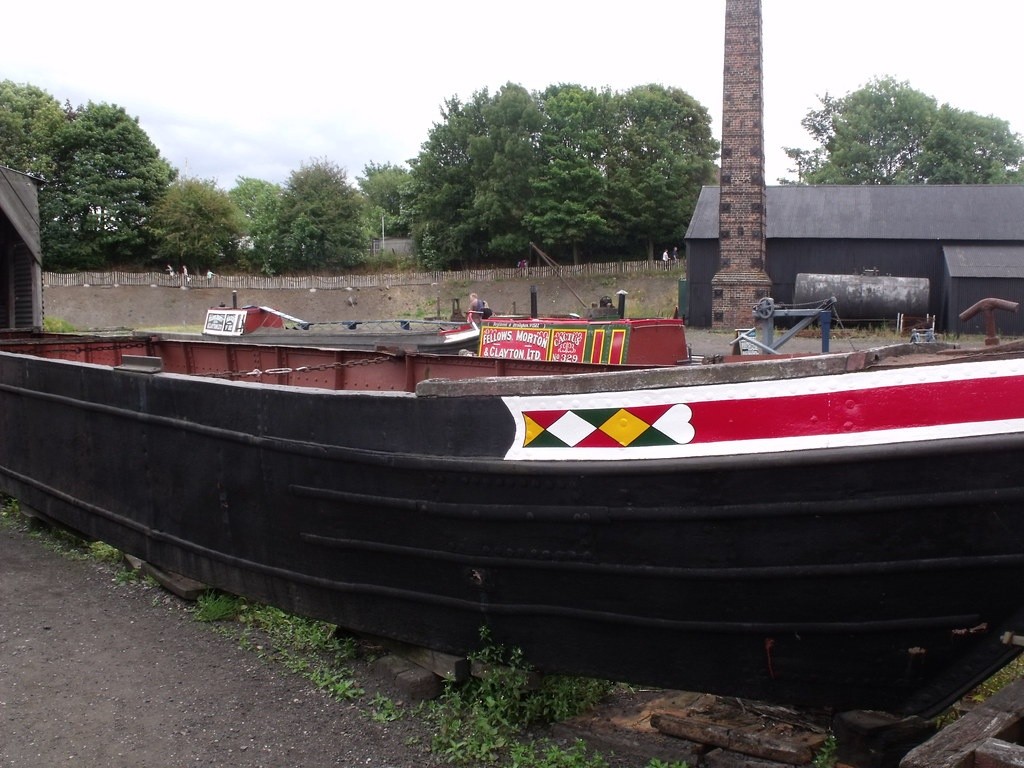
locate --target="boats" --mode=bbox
[10,312,1023,722]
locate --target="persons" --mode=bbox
[662,247,680,270]
[469,293,488,311]
[521,260,527,268]
